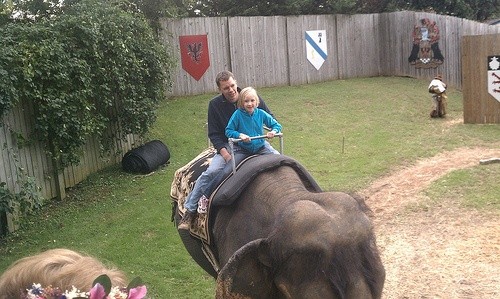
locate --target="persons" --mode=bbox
[177,70,277,231]
[197,87,283,214]
[0,249,147,299]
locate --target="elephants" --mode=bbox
[171,146,386,299]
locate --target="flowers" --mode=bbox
[23,274,148,299]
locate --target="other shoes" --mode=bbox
[197,195,209,214]
[177,210,196,230]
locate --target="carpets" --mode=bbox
[171,144,261,245]
